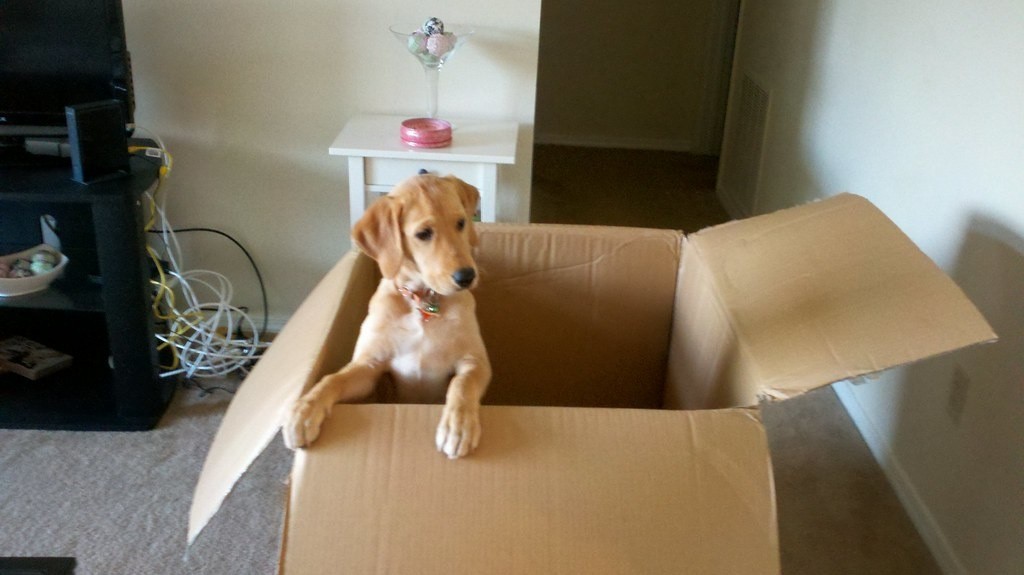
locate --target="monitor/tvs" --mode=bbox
[0,0,135,143]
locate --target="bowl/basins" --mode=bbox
[0,243,70,299]
[400,118,453,149]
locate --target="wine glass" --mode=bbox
[391,23,475,119]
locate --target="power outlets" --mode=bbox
[947,365,969,428]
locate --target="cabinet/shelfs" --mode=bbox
[0,153,177,432]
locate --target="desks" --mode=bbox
[328,113,519,249]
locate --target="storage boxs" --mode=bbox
[186,190,1000,575]
[0,334,74,380]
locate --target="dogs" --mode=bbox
[283,173,491,461]
